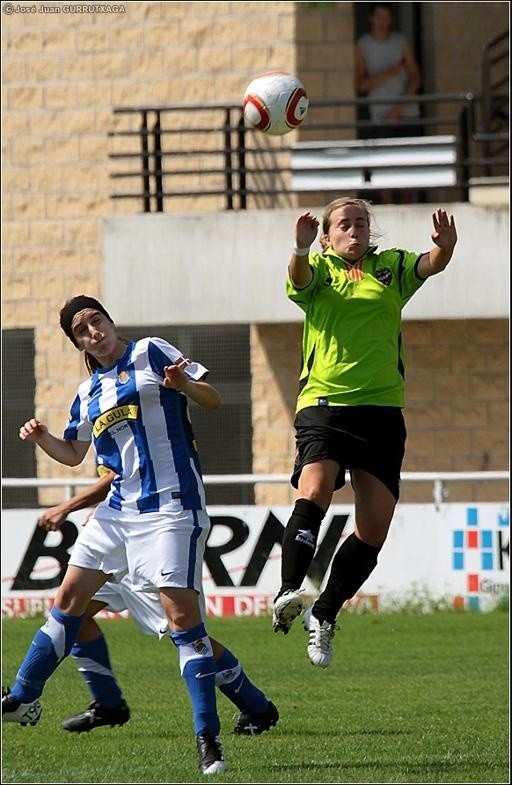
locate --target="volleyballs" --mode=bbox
[244,71,308,135]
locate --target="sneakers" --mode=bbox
[301,605,335,669]
[232,701,279,737]
[271,588,304,635]
[1,694,41,728]
[62,699,129,734]
[195,733,226,775]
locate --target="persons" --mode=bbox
[355,4,424,204]
[272,195,456,668]
[36,468,279,737]
[2,294,224,775]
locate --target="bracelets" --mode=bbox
[291,243,310,257]
[176,374,189,391]
